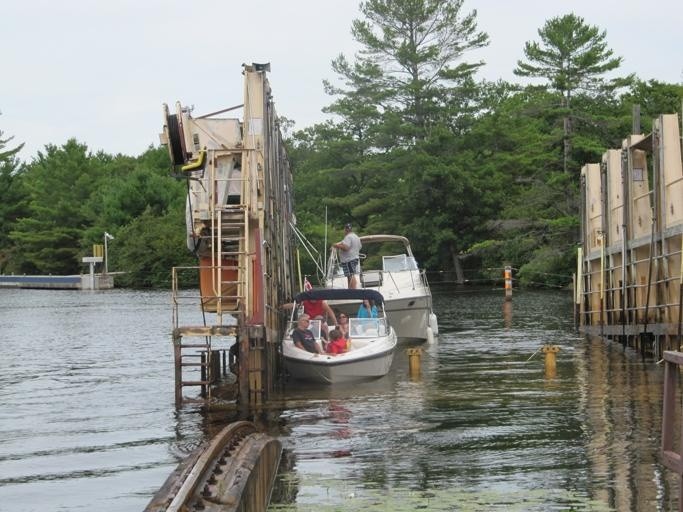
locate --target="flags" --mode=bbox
[304,279,312,291]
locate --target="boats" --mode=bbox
[277,285,401,387]
[323,230,442,349]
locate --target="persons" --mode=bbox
[310,316,329,349]
[338,331,350,352]
[332,222,362,289]
[292,315,321,353]
[279,296,338,326]
[336,312,349,338]
[325,330,346,354]
[357,299,377,318]
